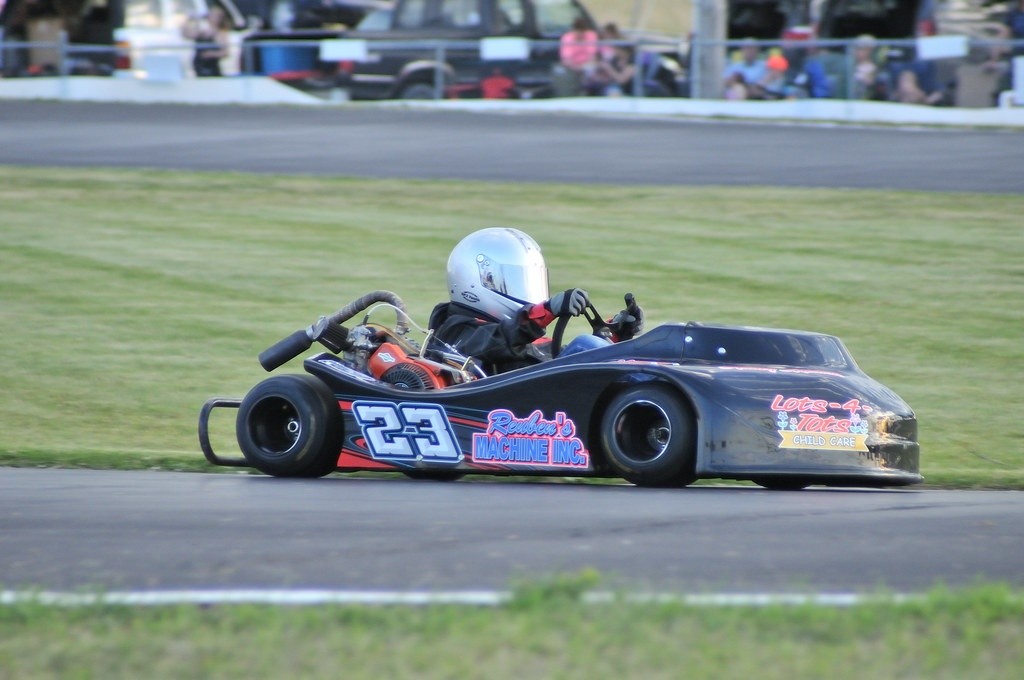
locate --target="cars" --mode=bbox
[60,0,253,78]
[318,0,679,98]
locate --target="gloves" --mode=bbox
[548,288,591,318]
[613,304,644,337]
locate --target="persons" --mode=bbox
[181,4,232,78]
[557,8,1024,110]
[422,227,645,380]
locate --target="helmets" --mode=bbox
[446,227,549,322]
